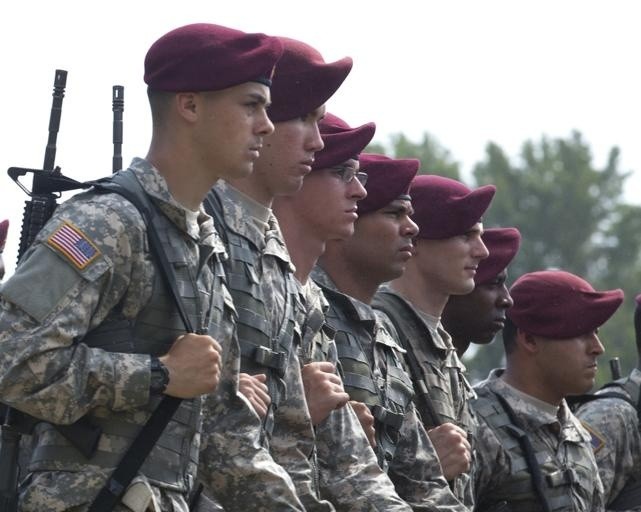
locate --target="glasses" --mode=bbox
[324,167,368,187]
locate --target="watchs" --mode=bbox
[150,356,170,398]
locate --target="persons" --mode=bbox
[473,271,625,512]
[443,226,521,512]
[375,175,495,512]
[578,296,641,512]
[224,36,335,512]
[319,154,468,512]
[274,114,410,512]
[1,23,304,512]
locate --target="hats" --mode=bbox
[506,271,625,340]
[356,153,420,214]
[410,174,496,239]
[474,227,521,285]
[312,111,376,170]
[266,36,353,122]
[144,24,284,93]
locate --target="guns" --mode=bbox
[1,70,101,511]
[113,86,122,175]
[609,357,621,380]
